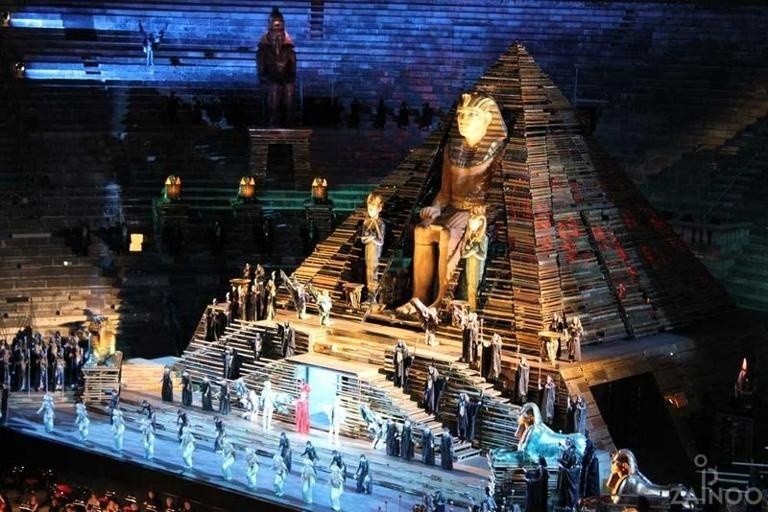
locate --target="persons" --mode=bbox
[395,90,508,329]
[0,262,586,511]
[255,5,297,129]
[459,205,487,312]
[359,193,385,305]
[142,32,155,66]
